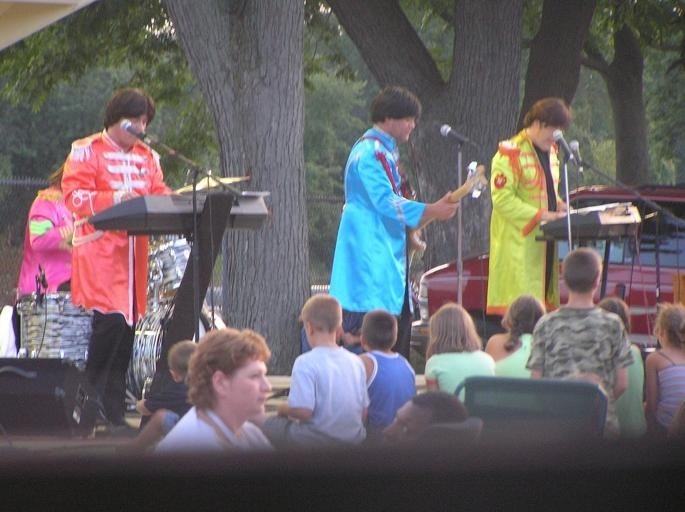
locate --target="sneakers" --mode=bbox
[100,405,129,429]
[72,407,96,439]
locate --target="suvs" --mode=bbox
[417,184,685,354]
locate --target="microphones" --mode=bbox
[568,138,583,165]
[440,124,475,150]
[36,263,50,289]
[119,118,156,152]
[551,129,575,161]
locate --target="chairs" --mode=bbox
[451,373,610,443]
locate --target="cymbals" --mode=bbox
[174,176,251,194]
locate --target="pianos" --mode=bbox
[538,201,642,240]
[89,192,268,235]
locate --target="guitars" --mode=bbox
[406,161,487,268]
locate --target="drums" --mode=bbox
[146,236,192,297]
[125,301,228,411]
[15,292,95,372]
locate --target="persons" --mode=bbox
[327,82,461,360]
[114,245,685,454]
[485,94,573,320]
[60,86,175,439]
[11,164,75,360]
